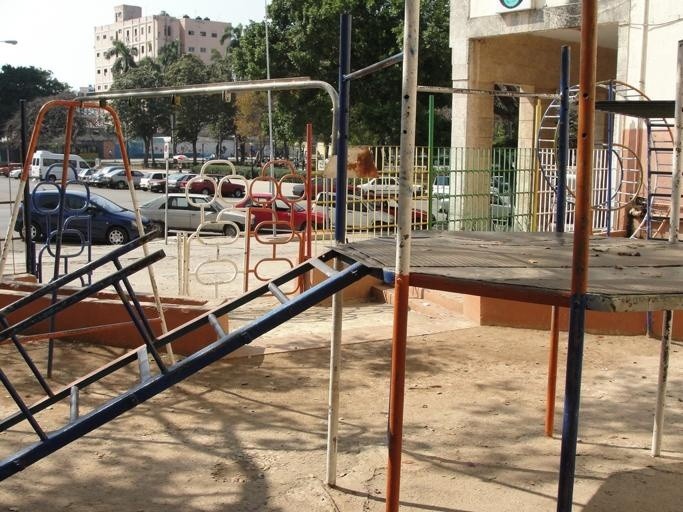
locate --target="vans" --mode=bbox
[30,150,94,180]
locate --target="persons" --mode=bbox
[107,149,112,158]
[249,142,256,158]
[220,146,228,160]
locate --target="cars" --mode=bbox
[0,165,23,179]
[431,172,515,225]
[13,189,154,246]
[132,193,255,236]
[76,166,245,198]
[234,194,329,233]
[292,176,435,231]
